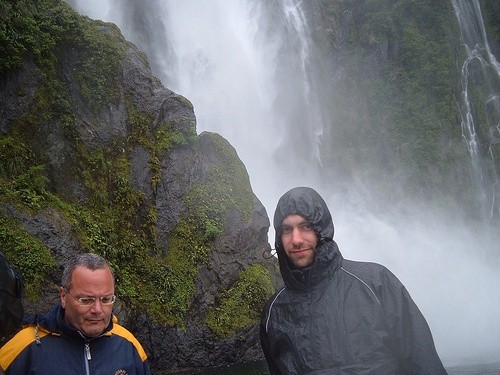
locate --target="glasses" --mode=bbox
[67,289,116,307]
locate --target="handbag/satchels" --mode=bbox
[0,250,24,337]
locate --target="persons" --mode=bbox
[0,253,152,375]
[259,186,448,375]
